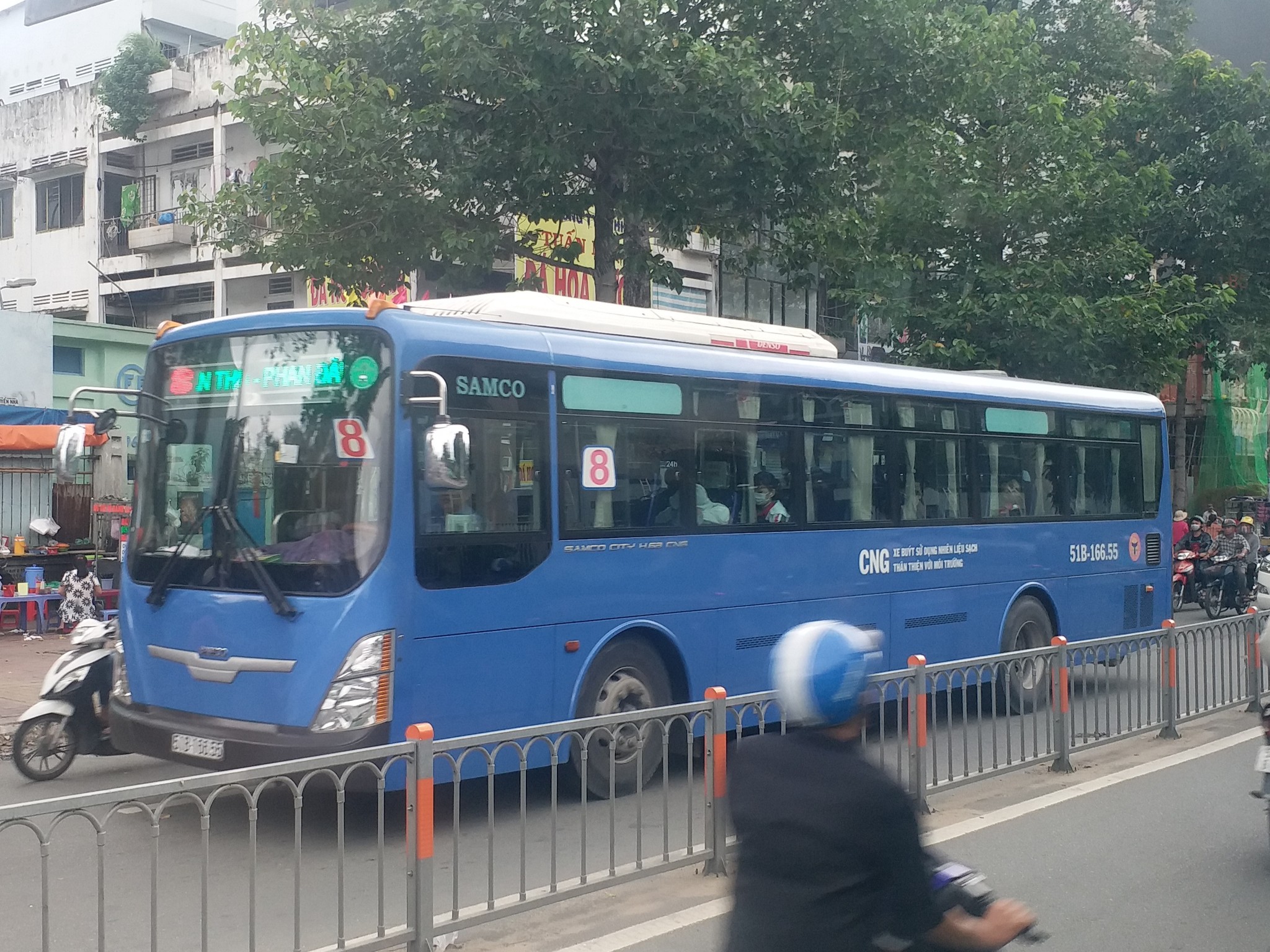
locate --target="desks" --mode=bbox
[0,549,106,584]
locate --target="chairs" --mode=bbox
[633,484,901,528]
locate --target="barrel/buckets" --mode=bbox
[23,564,44,588]
[2,585,16,597]
[14,534,26,554]
[18,582,28,595]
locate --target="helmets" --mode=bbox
[1221,519,1236,528]
[770,621,885,732]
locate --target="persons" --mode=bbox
[59,554,102,624]
[694,482,731,527]
[755,471,789,524]
[1000,479,1022,516]
[726,620,1038,952]
[1171,503,1261,608]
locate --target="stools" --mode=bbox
[0,590,120,628]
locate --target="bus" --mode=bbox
[55,287,1174,809]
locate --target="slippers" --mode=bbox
[1199,593,1206,600]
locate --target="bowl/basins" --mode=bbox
[52,588,58,594]
[55,545,70,552]
[85,555,104,568]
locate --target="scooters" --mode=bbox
[12,617,136,783]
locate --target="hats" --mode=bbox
[1237,516,1254,528]
[1173,510,1188,521]
[1190,515,1205,524]
[1207,503,1214,510]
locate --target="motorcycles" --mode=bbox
[1173,543,1207,613]
[1197,543,1269,620]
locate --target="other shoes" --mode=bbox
[1243,592,1250,602]
[1249,589,1254,596]
[56,627,65,635]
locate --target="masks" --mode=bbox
[1191,524,1201,531]
[754,492,773,506]
[1241,525,1248,534]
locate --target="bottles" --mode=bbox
[35,579,46,594]
